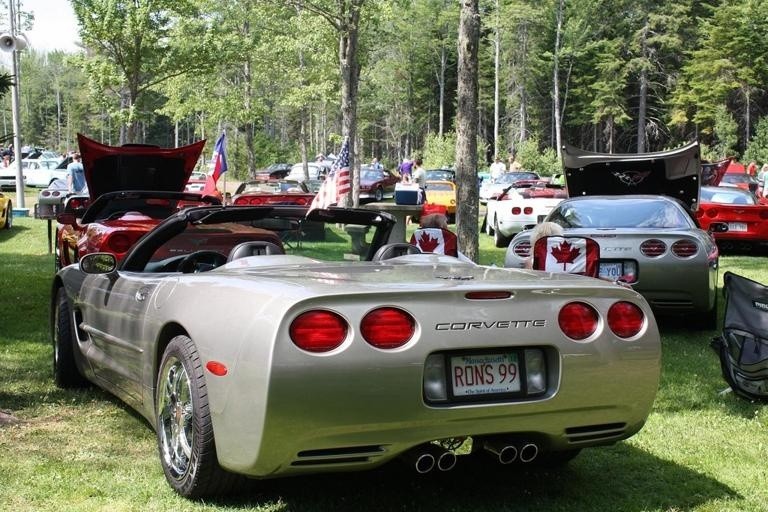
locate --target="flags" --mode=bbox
[305,139,351,219]
[201,134,227,200]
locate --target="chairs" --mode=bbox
[226,240,285,265]
[372,242,421,262]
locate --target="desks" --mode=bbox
[365,203,423,244]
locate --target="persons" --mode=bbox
[489,156,506,178]
[748,161,768,199]
[508,155,524,172]
[396,158,426,205]
[526,222,563,270]
[419,214,471,262]
[364,164,378,180]
[371,157,382,170]
[67,152,86,195]
[0,143,15,167]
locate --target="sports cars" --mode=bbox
[51,205,665,498]
[507,194,719,329]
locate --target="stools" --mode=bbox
[344,224,372,260]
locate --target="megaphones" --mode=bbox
[0,35,27,53]
